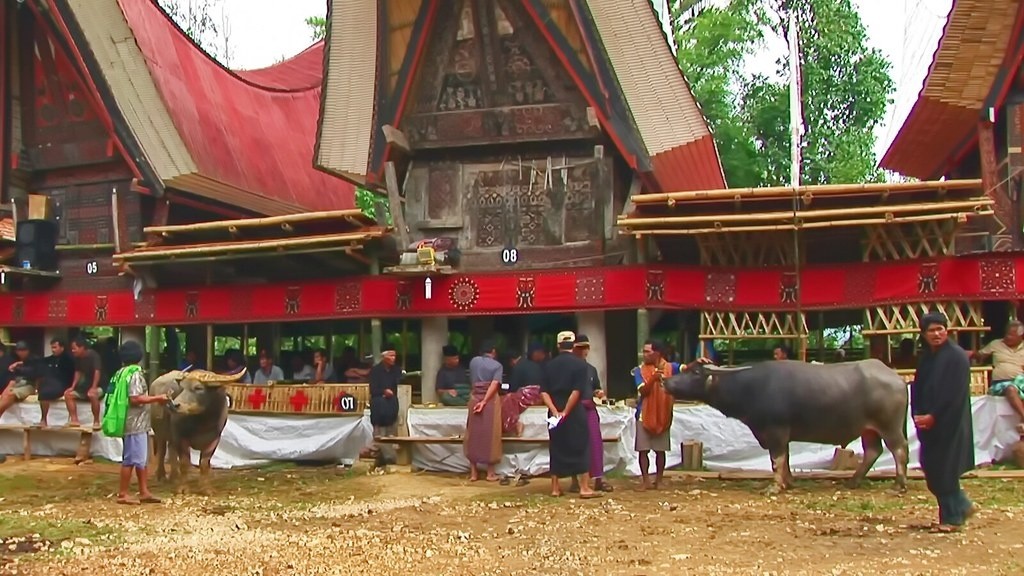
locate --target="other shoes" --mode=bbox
[388,434,395,438]
[470,472,479,481]
[117,497,141,504]
[487,475,499,481]
[595,481,613,491]
[373,435,381,439]
[64,421,80,427]
[92,420,101,430]
[140,495,162,503]
[570,484,579,492]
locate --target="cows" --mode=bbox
[149,366,248,498]
[663,359,909,494]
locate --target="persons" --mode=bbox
[634,340,713,492]
[834,348,847,362]
[966,321,1024,436]
[912,313,982,533]
[163,326,373,384]
[117,340,170,505]
[371,343,406,437]
[893,338,918,370]
[0,337,110,430]
[774,344,790,360]
[540,331,614,499]
[434,340,553,437]
[463,342,503,482]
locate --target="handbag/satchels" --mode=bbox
[644,381,672,433]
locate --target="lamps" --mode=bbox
[417,246,435,265]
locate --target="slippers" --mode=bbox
[929,524,963,533]
[551,489,562,497]
[965,503,981,519]
[515,477,529,487]
[580,490,607,497]
[499,476,509,485]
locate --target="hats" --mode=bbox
[443,345,458,355]
[16,341,28,349]
[380,344,395,352]
[557,331,575,343]
[527,343,544,355]
[574,335,589,347]
[920,312,946,328]
